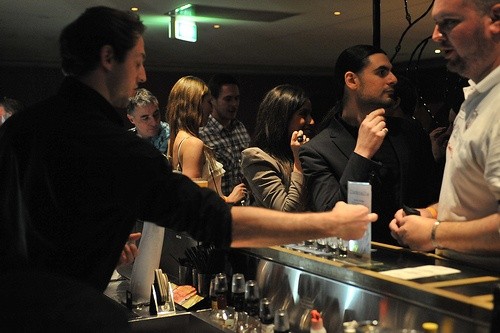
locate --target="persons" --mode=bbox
[127,88,170,157]
[298,45,438,247]
[241,85,315,212]
[165,76,248,204]
[0,6,378,333]
[385,78,417,120]
[198,74,251,197]
[429,100,465,186]
[389,0,500,258]
[0,96,20,125]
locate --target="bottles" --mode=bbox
[210,273,438,333]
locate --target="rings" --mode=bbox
[297,136,303,142]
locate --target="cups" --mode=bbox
[192,267,208,295]
[304,236,349,258]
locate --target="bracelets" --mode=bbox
[431,221,441,247]
[426,207,438,219]
